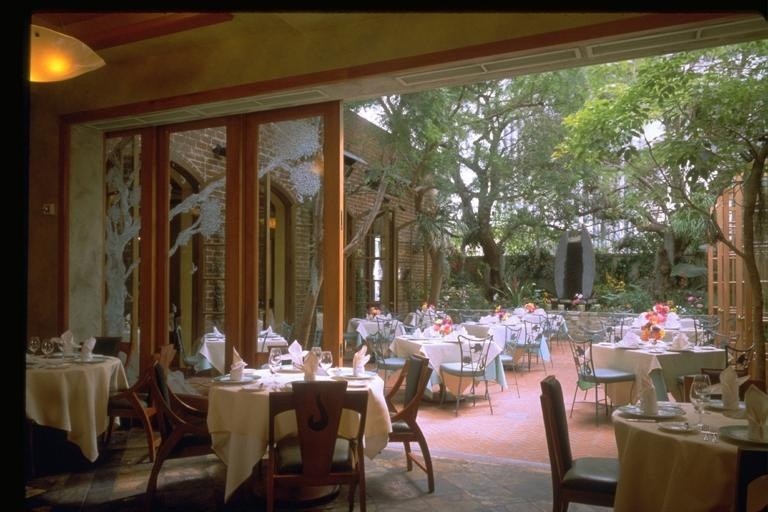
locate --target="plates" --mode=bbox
[475,312,544,325]
[622,319,682,330]
[335,368,376,379]
[717,424,768,445]
[706,399,745,411]
[615,403,688,419]
[241,382,268,392]
[657,421,698,433]
[598,334,715,354]
[75,357,105,364]
[214,374,262,385]
[404,326,474,343]
[339,378,369,388]
[203,331,282,343]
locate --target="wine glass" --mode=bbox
[24,336,74,368]
[265,345,333,385]
[688,374,711,431]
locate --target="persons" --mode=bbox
[170,305,183,369]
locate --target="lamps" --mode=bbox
[28,24,107,84]
